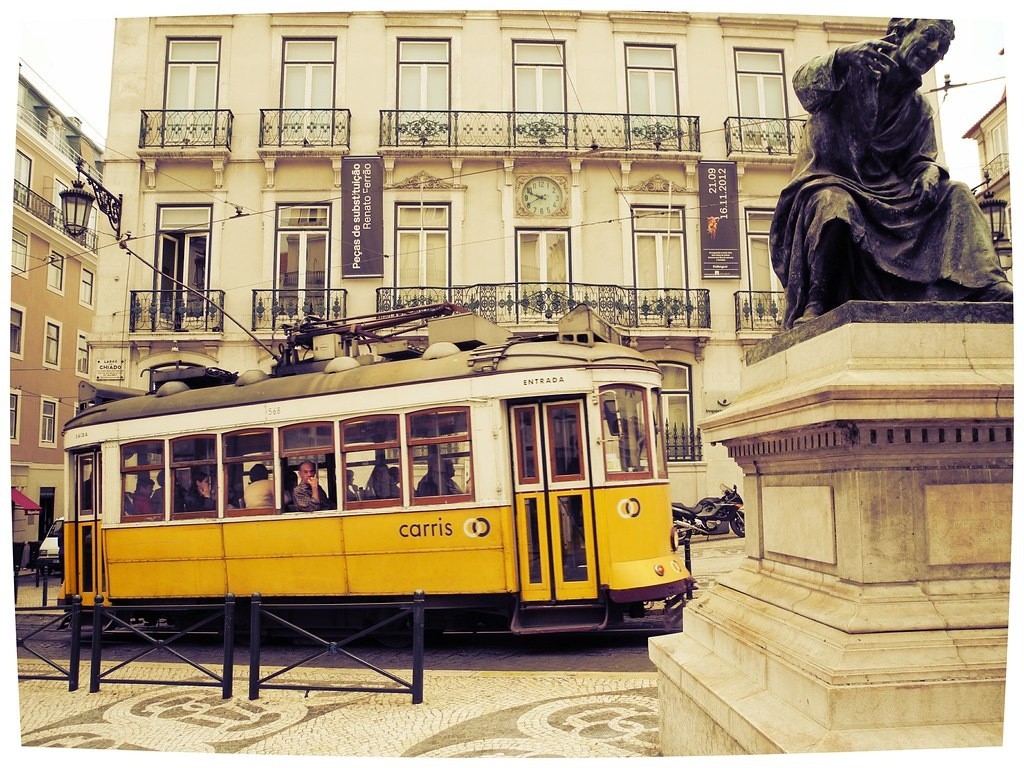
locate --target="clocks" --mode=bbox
[516,173,569,220]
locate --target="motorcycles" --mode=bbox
[670,483,745,544]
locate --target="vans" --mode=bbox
[37,516,65,575]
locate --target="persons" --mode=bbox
[80,468,98,543]
[344,460,403,500]
[57,518,65,582]
[293,459,332,513]
[132,477,155,514]
[153,467,186,511]
[184,471,216,511]
[417,453,447,495]
[767,18,1012,338]
[243,464,276,508]
[282,468,299,512]
[442,458,465,495]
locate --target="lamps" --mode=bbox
[58,159,124,240]
[971,173,1008,245]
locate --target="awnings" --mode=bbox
[12,486,44,515]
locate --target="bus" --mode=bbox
[56,297,698,649]
